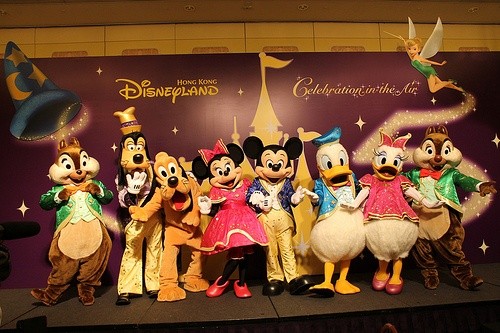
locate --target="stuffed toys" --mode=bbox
[400,124,498,289]
[114,107,163,306]
[192,138,270,298]
[341,128,446,294]
[243,136,314,296]
[30,137,114,307]
[302,127,366,295]
[129,152,210,302]
